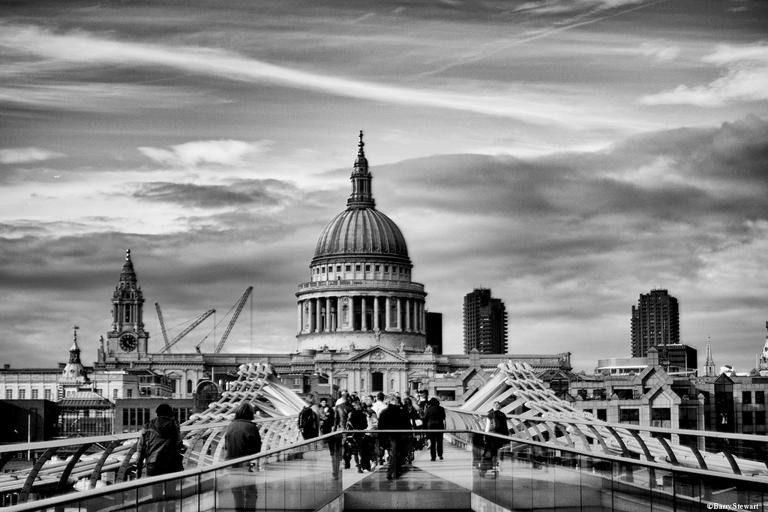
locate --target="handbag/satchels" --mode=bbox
[489,418,496,431]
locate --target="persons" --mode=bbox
[298,388,446,481]
[223,403,262,512]
[481,401,510,467]
[136,403,187,511]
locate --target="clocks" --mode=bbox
[119,333,138,353]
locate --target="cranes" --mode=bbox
[155,286,253,353]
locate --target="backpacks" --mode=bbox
[296,403,318,438]
